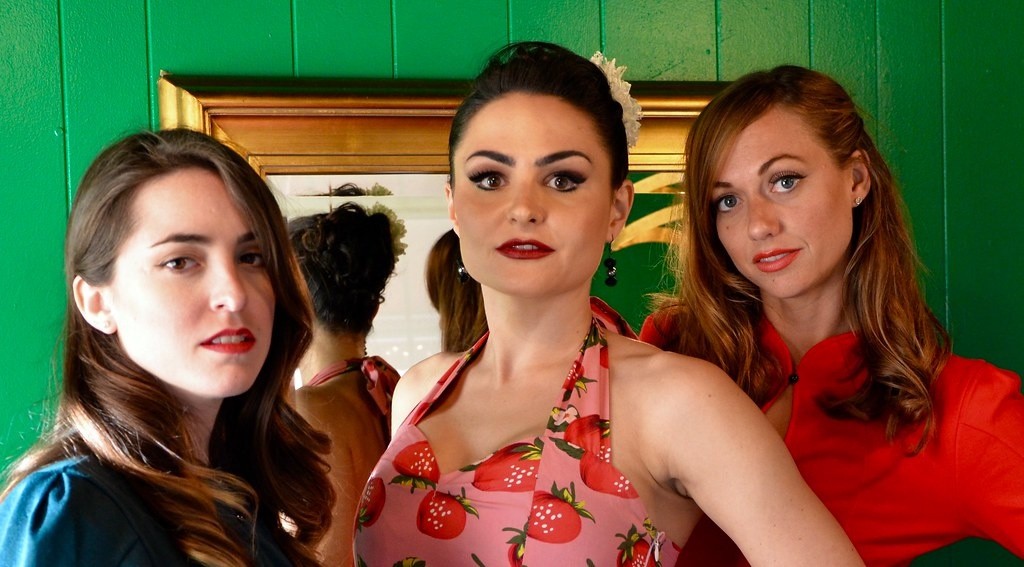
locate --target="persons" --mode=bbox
[636,64,1024,567]
[423,228,489,353]
[288,202,408,567]
[0,128,334,567]
[352,41,868,567]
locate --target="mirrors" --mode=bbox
[156,74,733,567]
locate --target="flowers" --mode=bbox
[365,201,408,265]
[590,51,643,149]
[364,181,392,196]
